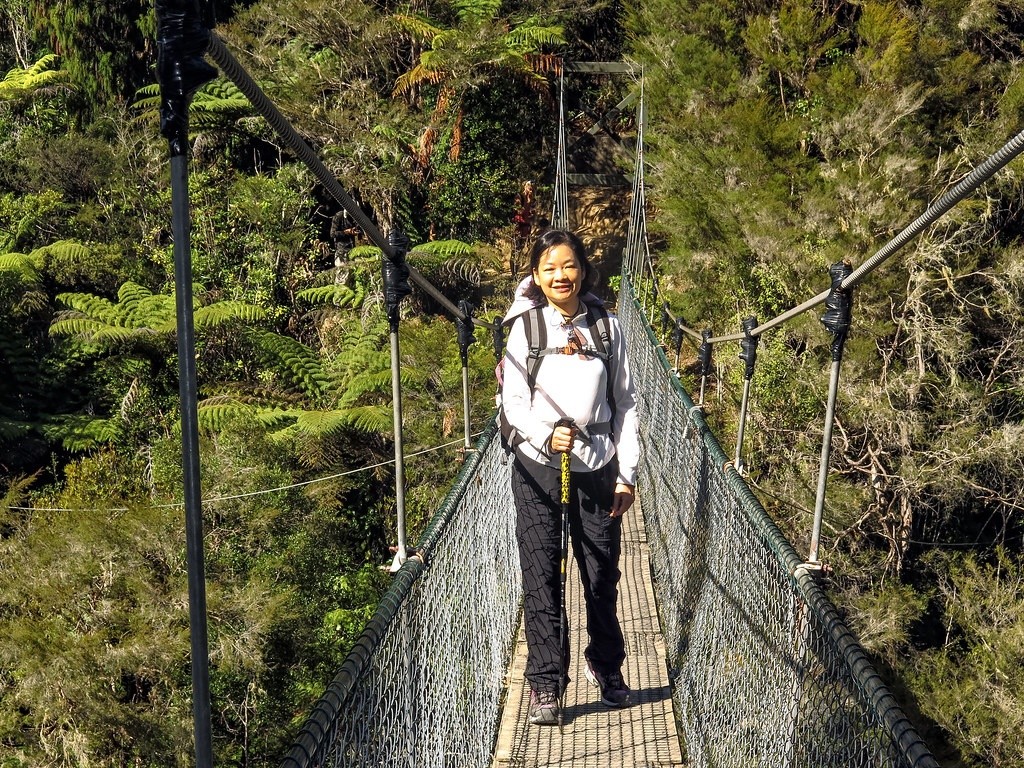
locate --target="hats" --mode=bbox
[502,274,601,327]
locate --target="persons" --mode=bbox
[501,230,642,726]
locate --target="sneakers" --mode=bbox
[528,688,563,725]
[584,660,631,707]
